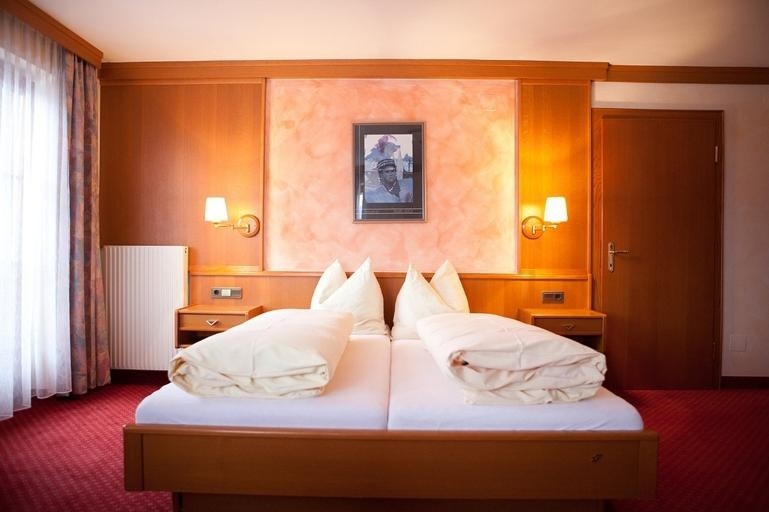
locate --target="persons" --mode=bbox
[376,159,400,196]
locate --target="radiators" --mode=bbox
[100,245,190,373]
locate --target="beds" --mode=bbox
[123,309,660,511]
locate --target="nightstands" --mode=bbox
[517,308,608,355]
[172,304,262,356]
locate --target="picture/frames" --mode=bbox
[352,121,426,223]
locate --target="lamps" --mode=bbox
[521,196,569,240]
[204,196,261,238]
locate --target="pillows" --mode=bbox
[309,255,469,338]
